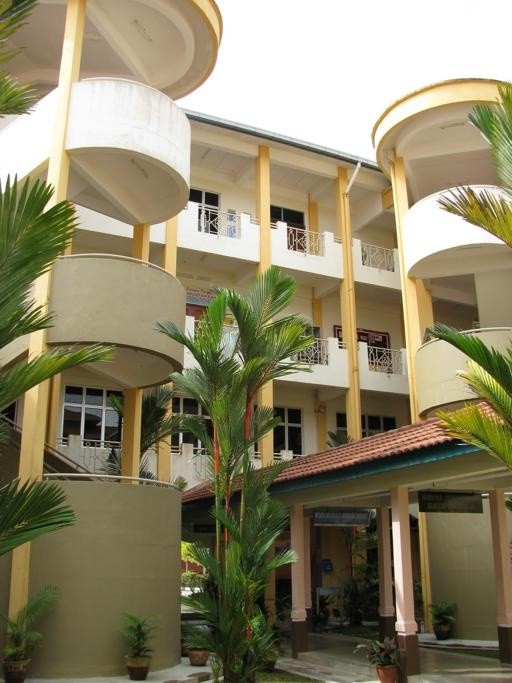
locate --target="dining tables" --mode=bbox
[351,635,401,682]
[182,628,213,666]
[426,598,457,640]
[121,610,157,679]
[1,582,61,682]
